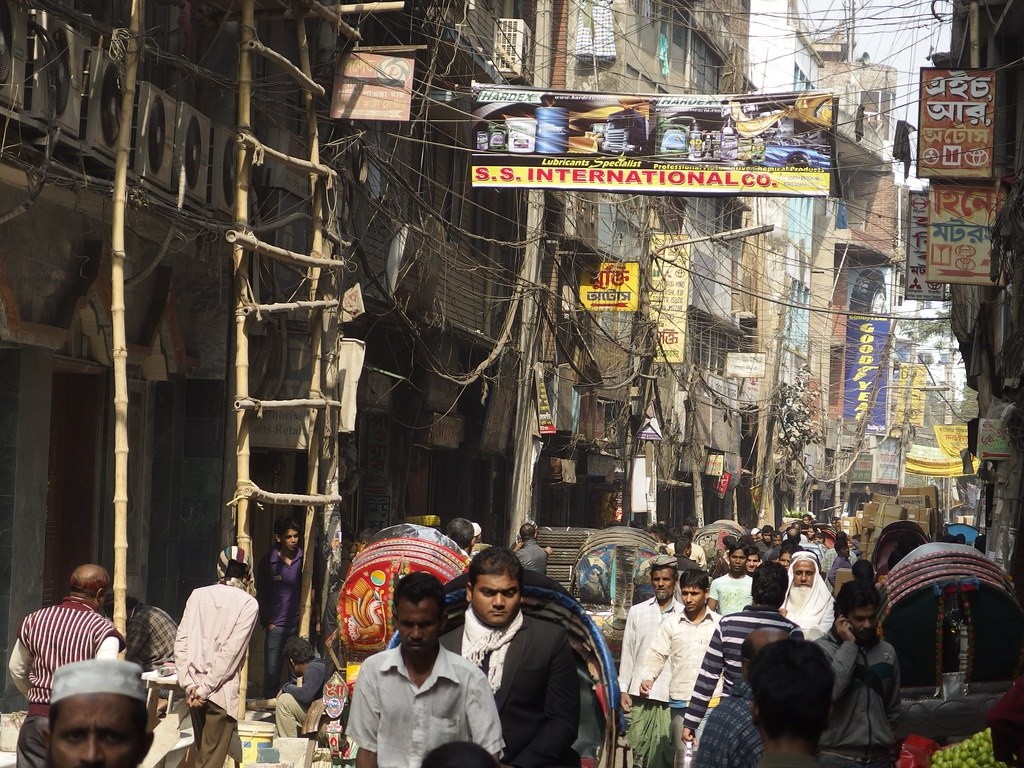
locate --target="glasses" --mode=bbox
[279,534,299,539]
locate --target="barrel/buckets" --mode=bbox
[942,671,969,701]
[0,713,27,751]
[228,720,276,768]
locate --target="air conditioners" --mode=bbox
[24,7,80,139]
[0,0,26,112]
[78,43,129,166]
[491,17,533,77]
[171,97,209,204]
[210,123,241,212]
[129,79,173,193]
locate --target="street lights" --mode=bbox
[841,386,950,513]
[621,224,774,526]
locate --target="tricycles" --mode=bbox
[301,629,360,768]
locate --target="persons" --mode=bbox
[9,564,127,768]
[346,571,506,768]
[618,555,685,768]
[447,518,482,555]
[707,543,753,615]
[355,528,379,550]
[510,520,553,575]
[748,640,836,768]
[276,638,326,738]
[315,560,352,660]
[812,581,901,768]
[174,546,259,768]
[256,516,315,698]
[694,627,793,768]
[438,546,580,768]
[708,514,875,581]
[778,551,836,641]
[102,589,178,672]
[630,519,708,570]
[44,659,155,768]
[639,569,723,768]
[681,560,804,749]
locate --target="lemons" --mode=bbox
[930,728,1008,768]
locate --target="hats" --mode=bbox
[470,522,482,537]
[49,658,146,702]
[751,527,760,535]
[648,554,677,569]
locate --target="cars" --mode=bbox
[799,543,826,558]
